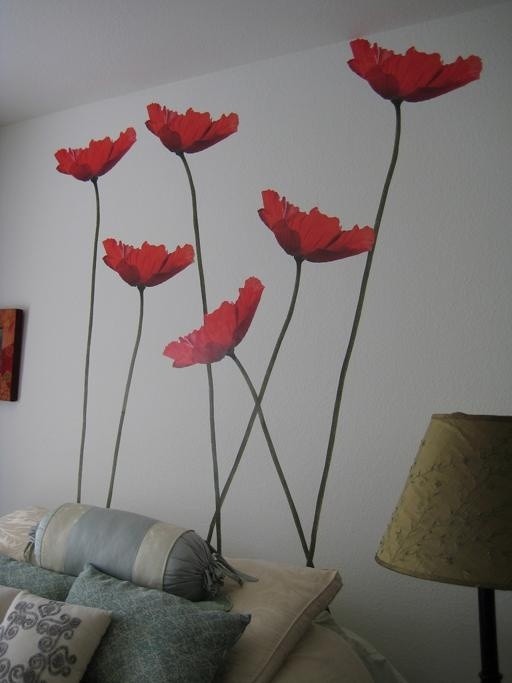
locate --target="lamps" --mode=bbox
[372,411,511,682]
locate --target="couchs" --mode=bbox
[1,503,413,683]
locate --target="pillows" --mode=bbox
[0,558,77,602]
[30,506,261,602]
[2,591,114,682]
[65,559,253,682]
[1,586,25,619]
[209,558,344,681]
